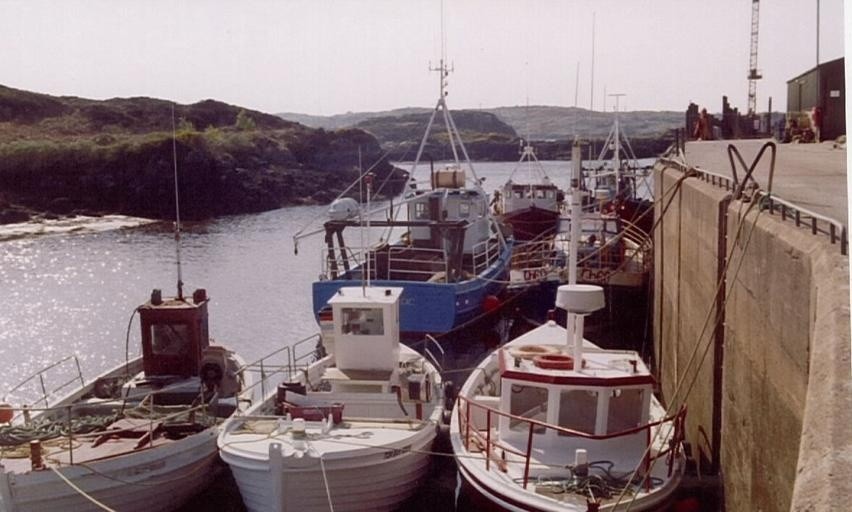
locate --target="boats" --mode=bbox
[490,13,655,333]
[442,152,694,512]
[218,143,455,510]
[291,0,514,353]
[0,107,239,511]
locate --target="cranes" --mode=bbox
[747,0,764,131]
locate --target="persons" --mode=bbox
[580,234,596,260]
[811,106,823,143]
[697,108,709,140]
